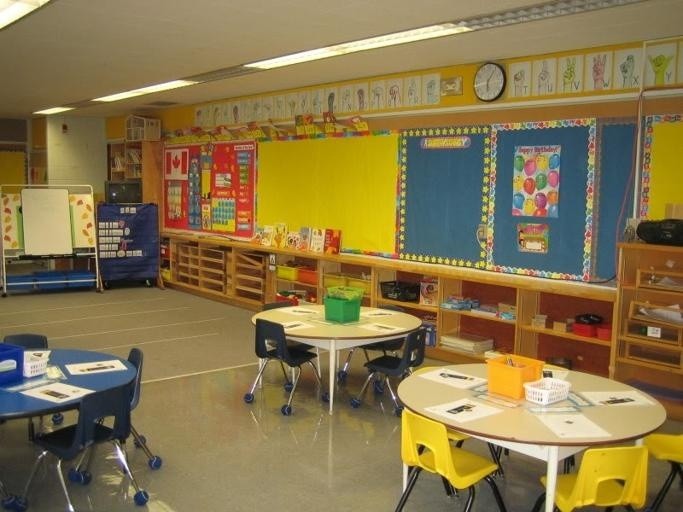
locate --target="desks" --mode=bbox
[397,361,667,510]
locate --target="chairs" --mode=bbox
[0,334,161,510]
[642,433,681,510]
[530,443,649,511]
[393,408,508,510]
[410,366,505,477]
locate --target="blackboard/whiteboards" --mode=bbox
[0,192,96,249]
[21,188,73,256]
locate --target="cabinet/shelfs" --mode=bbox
[607,236,681,419]
[158,234,618,380]
[106,142,163,232]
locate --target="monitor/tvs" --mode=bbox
[105,180,143,204]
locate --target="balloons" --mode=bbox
[512,152,560,217]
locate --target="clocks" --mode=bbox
[471,62,506,102]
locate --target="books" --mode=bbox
[110,149,140,179]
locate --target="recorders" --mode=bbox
[637,218,683,246]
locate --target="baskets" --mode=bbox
[24,358,49,377]
[523,377,571,405]
[381,281,419,301]
